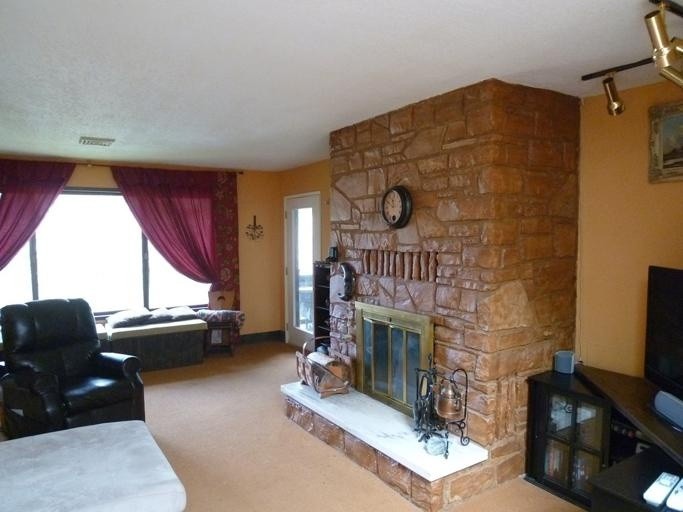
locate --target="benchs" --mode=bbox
[95,318,208,373]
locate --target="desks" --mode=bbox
[0,419,187,512]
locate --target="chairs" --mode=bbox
[201,289,236,359]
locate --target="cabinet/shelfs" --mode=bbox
[311,260,330,355]
[522,362,682,511]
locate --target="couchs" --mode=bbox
[0,296,146,440]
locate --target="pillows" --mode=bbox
[105,305,199,330]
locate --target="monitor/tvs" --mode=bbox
[644,264,683,434]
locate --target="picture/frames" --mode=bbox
[644,96,682,188]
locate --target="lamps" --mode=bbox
[578,0,682,116]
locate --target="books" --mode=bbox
[541,394,602,497]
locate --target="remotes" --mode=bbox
[644,472,679,506]
[666,478,682,511]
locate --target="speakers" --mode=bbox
[554,351,575,375]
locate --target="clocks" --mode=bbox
[379,184,414,231]
